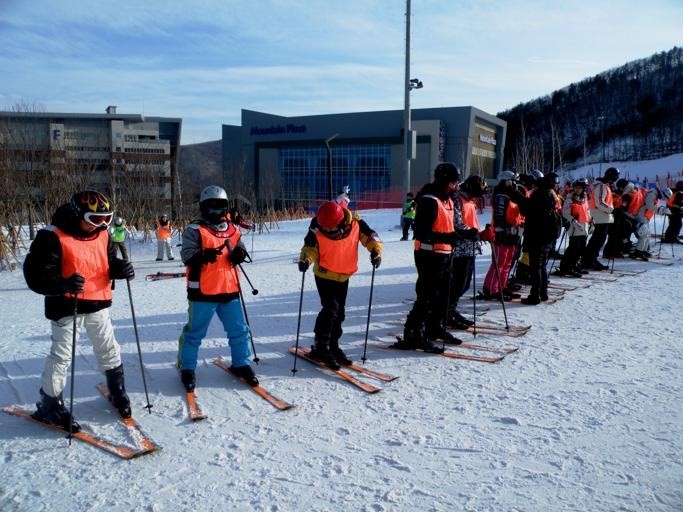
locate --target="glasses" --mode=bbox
[72,197,114,227]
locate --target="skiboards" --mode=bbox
[143,272,187,281]
[288,345,398,393]
[378,331,517,364]
[466,233,683,305]
[175,357,293,420]
[0,382,162,459]
[438,324,532,338]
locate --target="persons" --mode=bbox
[176,185,257,393]
[107,207,254,260]
[22,190,136,434]
[397,163,682,353]
[298,200,380,372]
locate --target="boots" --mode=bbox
[39,387,73,422]
[105,364,130,409]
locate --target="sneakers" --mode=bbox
[315,347,337,363]
[403,251,652,354]
[233,362,255,377]
[182,369,196,384]
[332,347,346,359]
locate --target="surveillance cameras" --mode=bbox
[410,80,423,88]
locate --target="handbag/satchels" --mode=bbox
[551,209,563,239]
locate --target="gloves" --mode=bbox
[120,260,134,279]
[442,232,461,244]
[204,247,222,263]
[370,252,381,268]
[464,228,477,239]
[299,261,308,271]
[229,247,246,263]
[64,274,85,293]
[480,228,496,242]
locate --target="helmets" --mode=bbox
[317,201,344,227]
[115,217,122,225]
[161,213,167,217]
[71,190,111,221]
[427,163,673,200]
[200,185,230,212]
